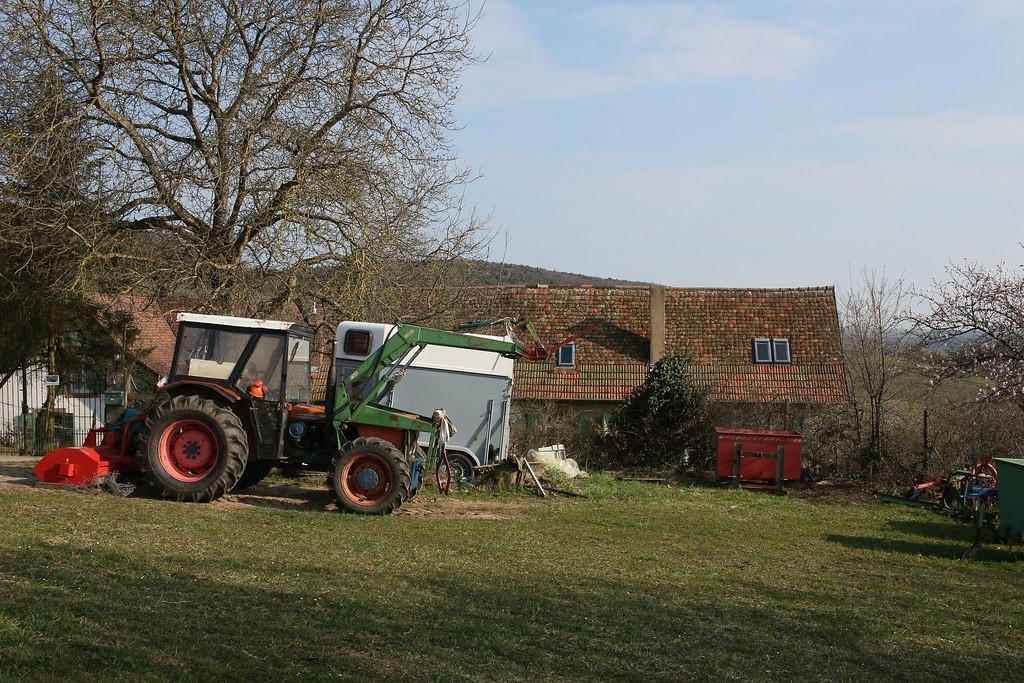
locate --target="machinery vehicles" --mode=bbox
[131,309,611,517]
